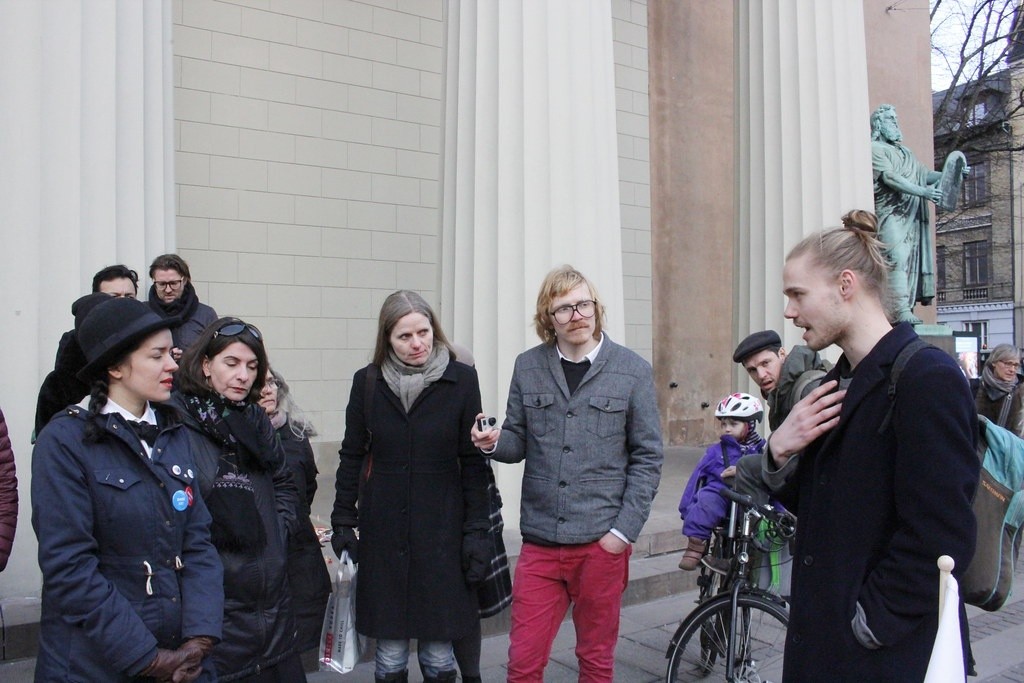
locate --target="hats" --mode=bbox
[733,330,782,363]
[448,338,474,367]
[77,297,183,383]
[72,292,112,333]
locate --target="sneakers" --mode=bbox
[701,554,732,576]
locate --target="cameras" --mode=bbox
[478,416,497,432]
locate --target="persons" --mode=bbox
[140,255,219,363]
[32,292,226,682]
[331,290,513,682]
[0,410,19,571]
[160,316,329,682]
[678,394,768,569]
[471,264,663,683]
[35,266,138,436]
[968,343,1024,442]
[701,330,833,576]
[870,105,972,322]
[759,209,983,683]
[258,367,320,516]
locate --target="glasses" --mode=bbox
[550,299,597,326]
[263,379,281,390]
[153,275,185,291]
[1000,359,1021,370]
[214,322,263,343]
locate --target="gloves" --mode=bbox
[172,636,216,683]
[138,646,203,683]
[460,529,493,591]
[330,517,359,563]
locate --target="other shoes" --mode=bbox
[679,537,706,571]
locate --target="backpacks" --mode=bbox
[878,340,1024,612]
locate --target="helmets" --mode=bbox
[714,392,764,424]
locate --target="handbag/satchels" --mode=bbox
[354,362,378,514]
[318,549,367,675]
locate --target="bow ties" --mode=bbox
[127,420,159,448]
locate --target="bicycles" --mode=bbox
[663,487,795,683]
